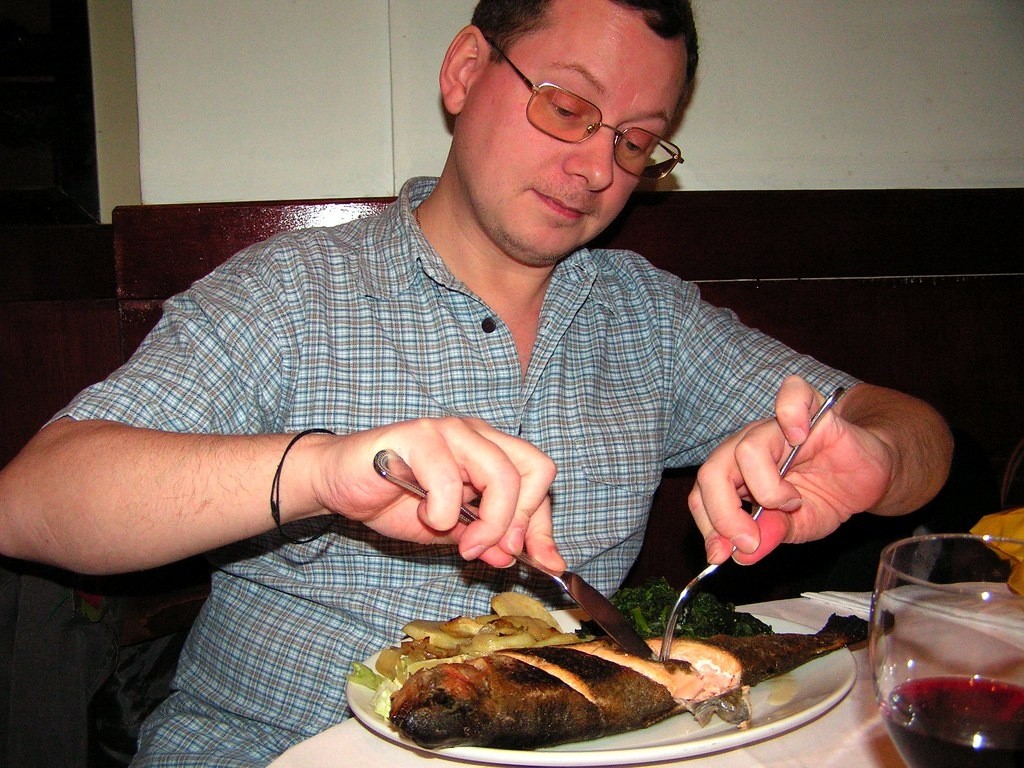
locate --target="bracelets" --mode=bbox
[269,427,341,546]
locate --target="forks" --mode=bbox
[658,386,848,663]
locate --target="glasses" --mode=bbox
[488,36,684,181]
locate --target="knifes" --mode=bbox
[372,448,655,661]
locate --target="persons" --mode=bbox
[0,0,954,766]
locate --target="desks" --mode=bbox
[263,584,1024,768]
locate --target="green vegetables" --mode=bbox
[575,577,772,639]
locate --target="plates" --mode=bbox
[345,609,859,766]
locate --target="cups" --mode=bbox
[869,533,1024,768]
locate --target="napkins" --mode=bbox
[799,591,1024,639]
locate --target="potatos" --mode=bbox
[375,594,564,675]
